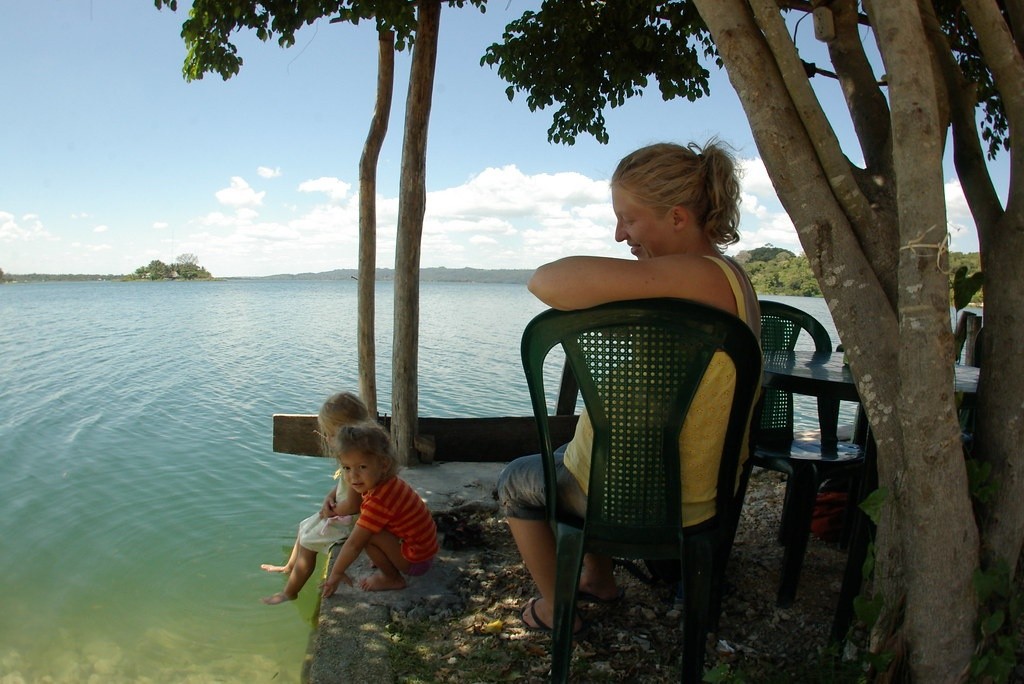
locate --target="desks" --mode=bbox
[760,350,979,647]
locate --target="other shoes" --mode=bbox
[443,523,488,551]
[432,512,467,532]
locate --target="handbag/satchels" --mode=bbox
[810,479,849,542]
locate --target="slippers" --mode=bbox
[580,586,625,607]
[520,598,590,641]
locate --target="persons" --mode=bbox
[262,392,387,605]
[498,143,763,636]
[319,425,440,599]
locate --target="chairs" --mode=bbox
[720,298,868,610]
[519,296,761,683]
